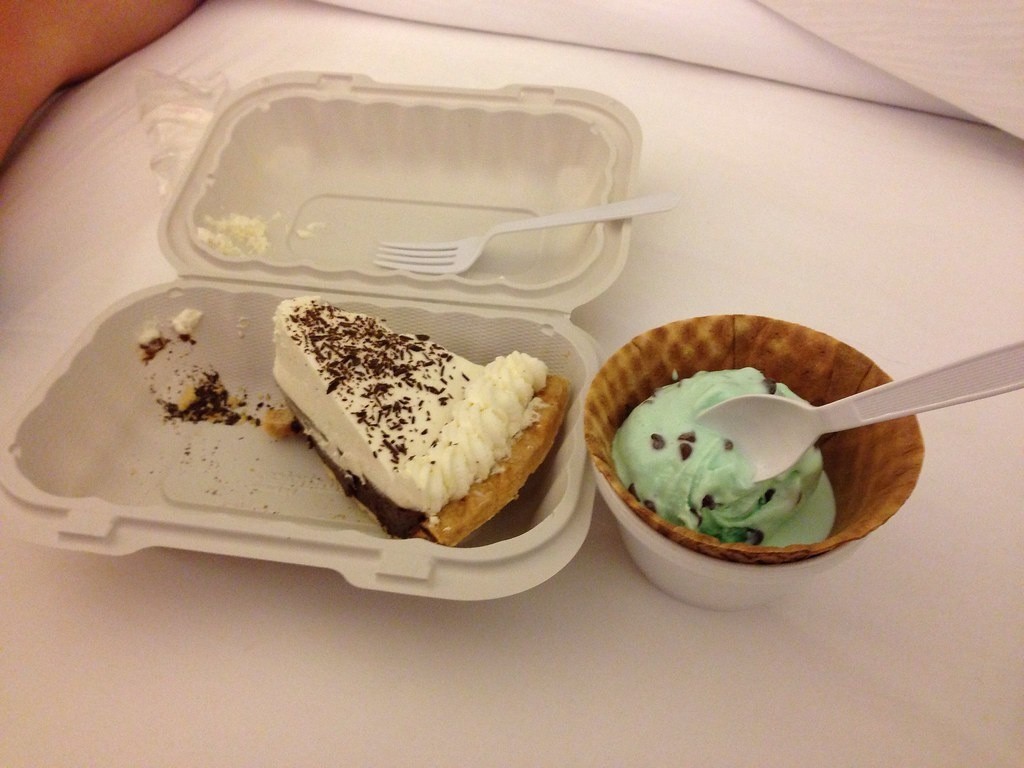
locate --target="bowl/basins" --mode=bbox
[1,67,649,602]
[593,436,867,611]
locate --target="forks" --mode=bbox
[371,194,671,273]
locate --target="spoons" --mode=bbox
[696,339,1024,484]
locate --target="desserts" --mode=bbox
[270,295,569,547]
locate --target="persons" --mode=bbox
[1,2,203,159]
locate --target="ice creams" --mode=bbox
[612,366,836,547]
